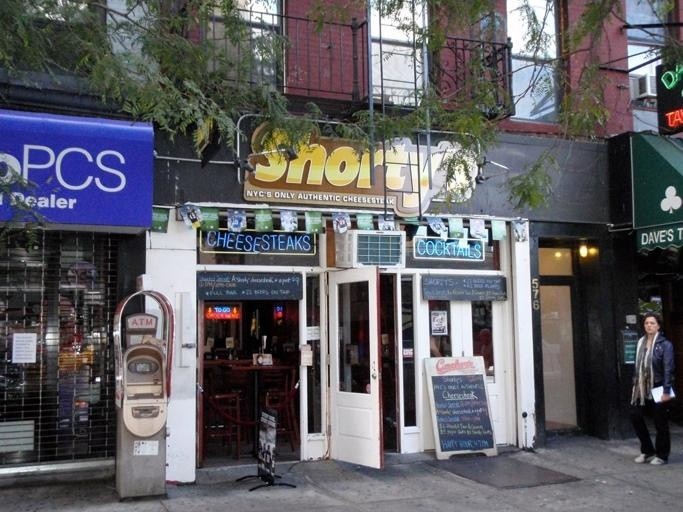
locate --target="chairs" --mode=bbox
[200,368,298,461]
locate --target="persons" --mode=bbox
[478,329,494,367]
[628,311,675,466]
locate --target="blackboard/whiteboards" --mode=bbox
[201,276,304,300]
[421,275,508,302]
[424,356,498,461]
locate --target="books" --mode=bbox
[650,384,676,404]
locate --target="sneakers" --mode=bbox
[634,451,668,465]
